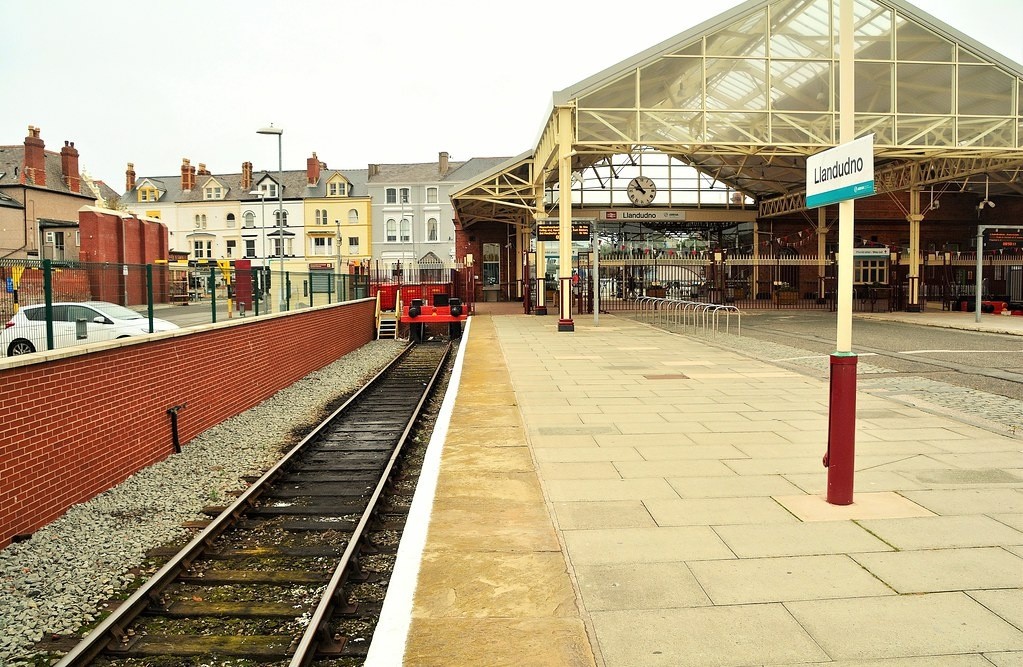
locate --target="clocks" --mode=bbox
[627,176,656,207]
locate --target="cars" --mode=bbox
[0,301,181,357]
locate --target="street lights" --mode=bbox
[250,191,267,314]
[256,127,284,301]
[402,199,406,285]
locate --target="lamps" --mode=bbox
[760,171,766,179]
[602,157,608,165]
[968,184,974,191]
[734,181,740,189]
[988,201,995,207]
[690,157,696,166]
[979,200,985,209]
[793,159,798,168]
